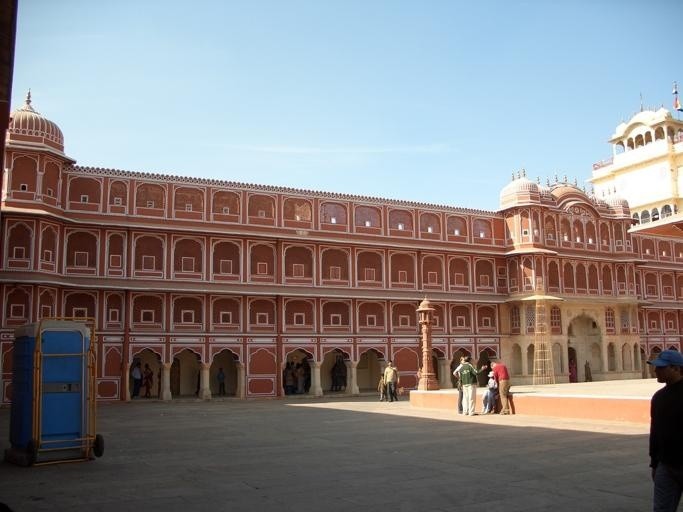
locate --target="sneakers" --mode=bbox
[459,409,517,416]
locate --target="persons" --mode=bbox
[377,373,388,402]
[451,355,464,413]
[330,355,340,391]
[459,356,487,416]
[215,366,227,396]
[481,371,498,414]
[335,356,347,390]
[383,359,400,402]
[130,361,143,400]
[645,348,683,512]
[140,362,154,398]
[490,360,512,415]
[283,359,311,395]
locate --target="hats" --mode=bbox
[487,370,494,378]
[645,349,683,368]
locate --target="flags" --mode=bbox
[672,95,682,113]
